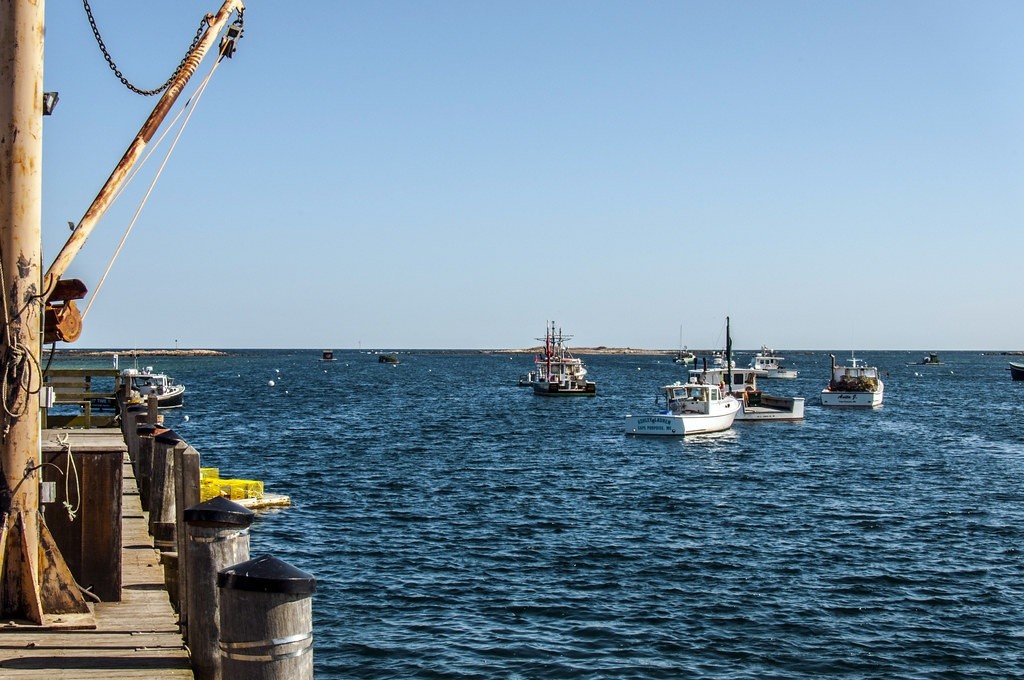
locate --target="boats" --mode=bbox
[84,344,186,410]
[378,355,401,364]
[818,347,884,406]
[317,348,339,361]
[1008,361,1024,381]
[519,320,597,396]
[905,352,946,366]
[623,314,806,437]
